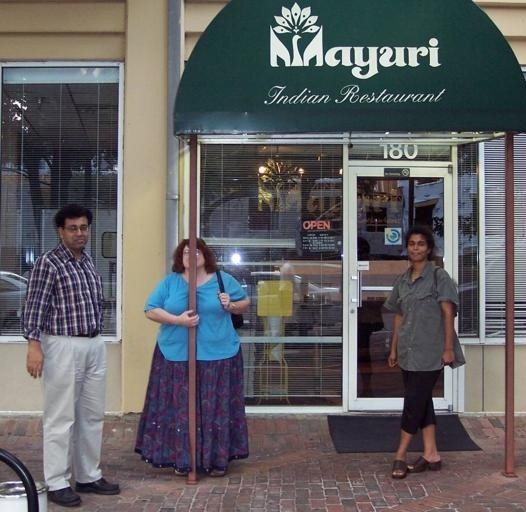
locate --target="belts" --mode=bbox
[70,330,100,338]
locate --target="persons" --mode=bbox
[379,225,463,481]
[133,235,252,479]
[19,203,122,507]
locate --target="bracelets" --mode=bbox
[231,302,238,312]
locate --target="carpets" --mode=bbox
[325,414,483,454]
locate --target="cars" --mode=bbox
[234,270,329,337]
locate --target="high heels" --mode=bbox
[407,456,442,472]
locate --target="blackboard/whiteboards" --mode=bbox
[299,214,342,260]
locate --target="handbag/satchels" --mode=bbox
[450,328,466,369]
[216,269,244,330]
[370,306,384,332]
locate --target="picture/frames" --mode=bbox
[357,176,414,261]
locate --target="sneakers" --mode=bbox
[75,477,120,495]
[206,468,226,477]
[174,469,187,476]
[47,486,81,507]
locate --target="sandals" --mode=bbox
[392,460,407,478]
